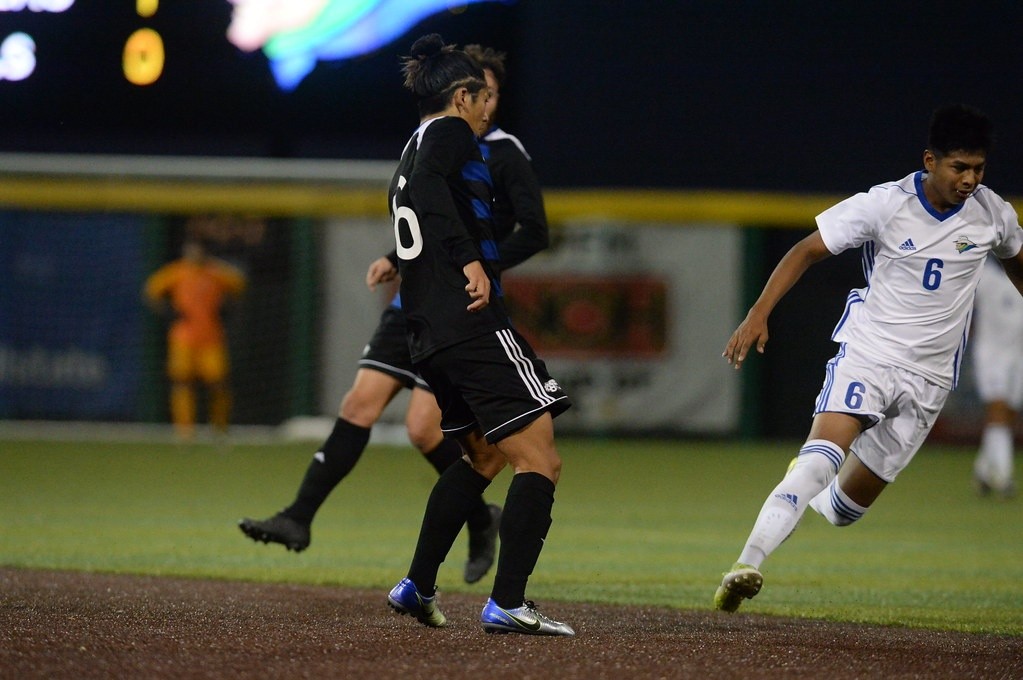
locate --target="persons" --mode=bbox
[711,103,1022,612]
[143,231,248,440]
[385,34,573,638]
[236,43,553,587]
[862,236,1023,499]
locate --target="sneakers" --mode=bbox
[785,456,799,478]
[239,511,311,552]
[464,504,501,583]
[388,575,446,628]
[714,566,764,615]
[480,598,576,636]
